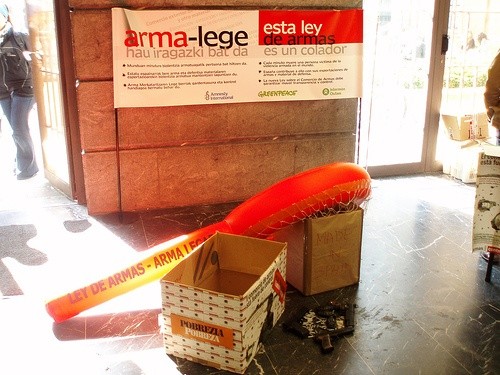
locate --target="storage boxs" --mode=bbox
[159,231,289,375]
[266,200,366,295]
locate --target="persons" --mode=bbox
[0,1,43,181]
[483,47,500,146]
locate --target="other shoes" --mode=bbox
[17,166,39,179]
